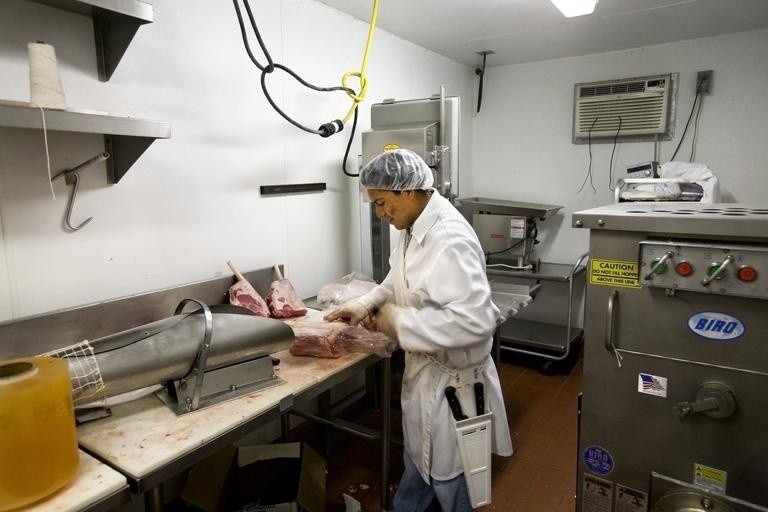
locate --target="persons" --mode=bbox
[320,145,517,510]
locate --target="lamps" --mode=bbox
[550,0,597,19]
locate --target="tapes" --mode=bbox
[0,357,80,512]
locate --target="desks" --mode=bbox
[8,443,131,512]
[76,308,392,512]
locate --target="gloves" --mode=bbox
[323,300,369,326]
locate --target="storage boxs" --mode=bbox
[180,434,330,510]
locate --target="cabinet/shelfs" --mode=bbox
[0,0,173,138]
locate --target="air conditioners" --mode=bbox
[572,73,673,139]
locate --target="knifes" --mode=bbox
[473,382,484,415]
[444,386,467,422]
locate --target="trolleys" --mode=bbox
[484,252,589,371]
